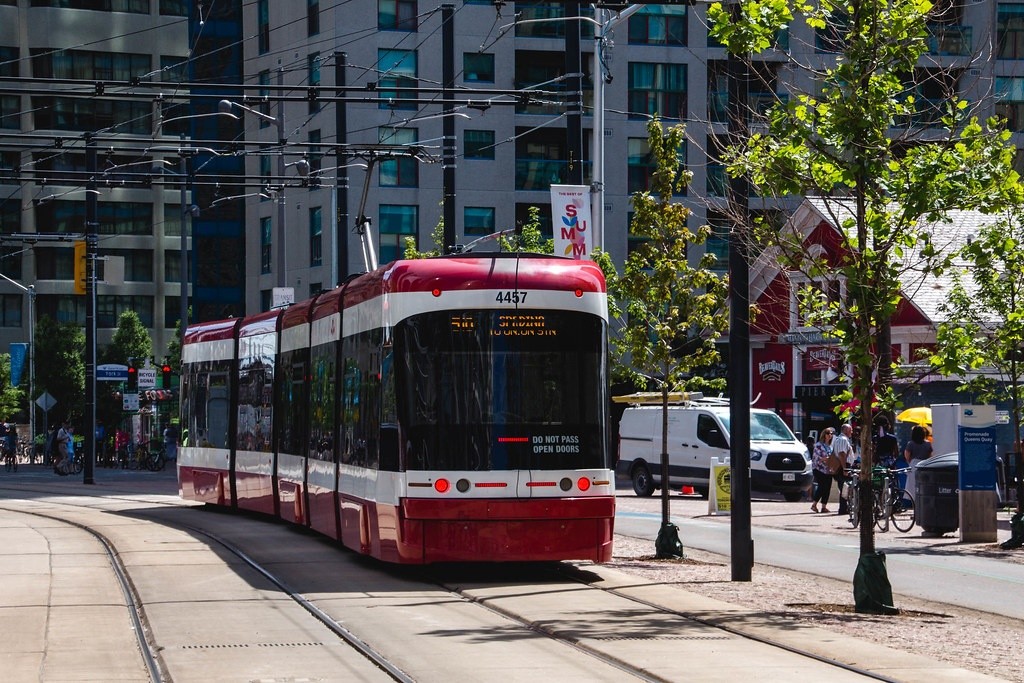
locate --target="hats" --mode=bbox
[911,422,932,435]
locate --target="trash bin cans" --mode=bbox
[914,453,1004,538]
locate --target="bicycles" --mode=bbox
[845,466,916,533]
[4,448,18,473]
[74,442,84,473]
[107,440,165,471]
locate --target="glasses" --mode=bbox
[826,432,834,435]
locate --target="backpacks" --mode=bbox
[51,428,67,452]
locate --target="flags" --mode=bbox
[550,187,592,260]
[10,343,28,388]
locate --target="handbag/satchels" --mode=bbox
[842,476,854,500]
[827,453,841,472]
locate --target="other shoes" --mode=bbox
[54,465,57,473]
[839,509,849,514]
[910,516,914,520]
[821,508,830,512]
[60,472,68,476]
[811,505,818,511]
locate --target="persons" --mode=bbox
[163,422,177,468]
[806,424,861,515]
[0,419,20,472]
[95,422,106,464]
[872,416,900,513]
[55,420,75,476]
[115,428,130,469]
[903,424,933,520]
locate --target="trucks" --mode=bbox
[618,391,814,501]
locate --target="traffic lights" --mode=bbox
[162,364,171,389]
[127,365,137,388]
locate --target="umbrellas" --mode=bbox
[896,407,932,427]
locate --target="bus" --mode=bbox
[175,249,617,565]
